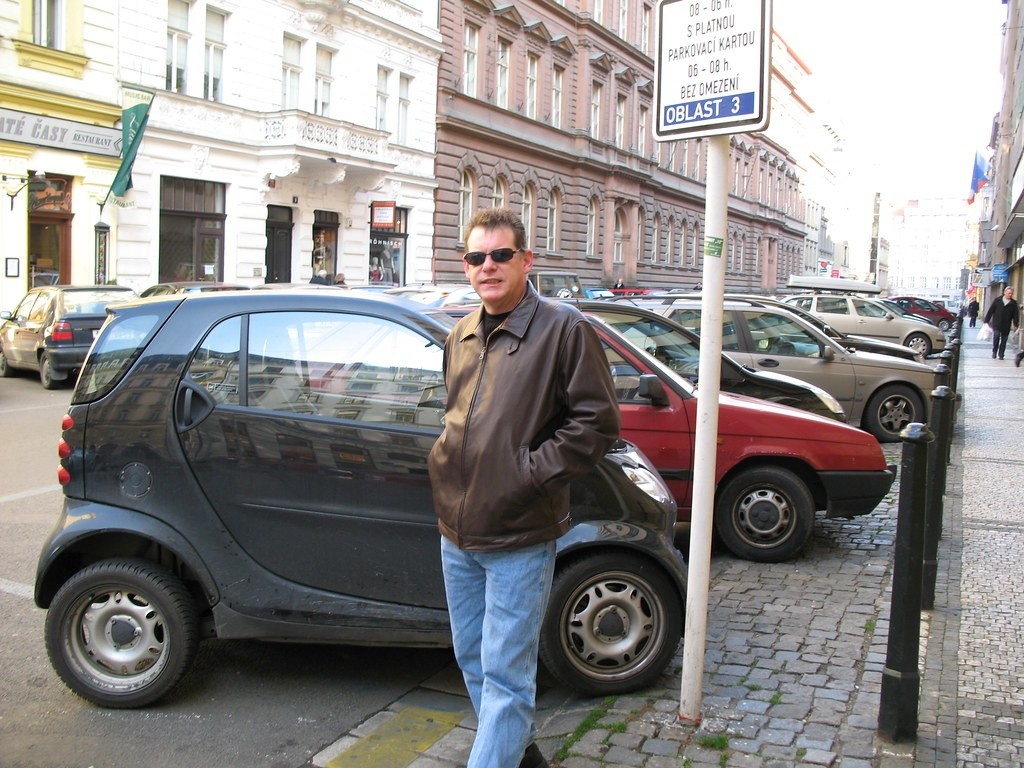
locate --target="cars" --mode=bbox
[556,295,850,430]
[252,281,483,322]
[299,306,899,565]
[32,288,688,713]
[526,271,587,301]
[137,279,251,297]
[0,284,142,390]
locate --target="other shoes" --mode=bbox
[998,353,1003,360]
[519,742,547,768]
[992,352,996,359]
[1014,354,1021,367]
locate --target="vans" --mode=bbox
[582,274,960,442]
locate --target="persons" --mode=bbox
[428,207,622,767]
[334,272,347,286]
[369,241,396,283]
[1015,352,1024,367]
[967,295,979,328]
[309,270,328,286]
[983,285,1020,360]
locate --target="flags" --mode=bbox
[967,149,993,205]
[113,103,150,197]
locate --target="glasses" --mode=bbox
[464,248,521,265]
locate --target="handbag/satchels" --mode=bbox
[977,323,993,342]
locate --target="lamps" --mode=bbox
[1,167,45,211]
